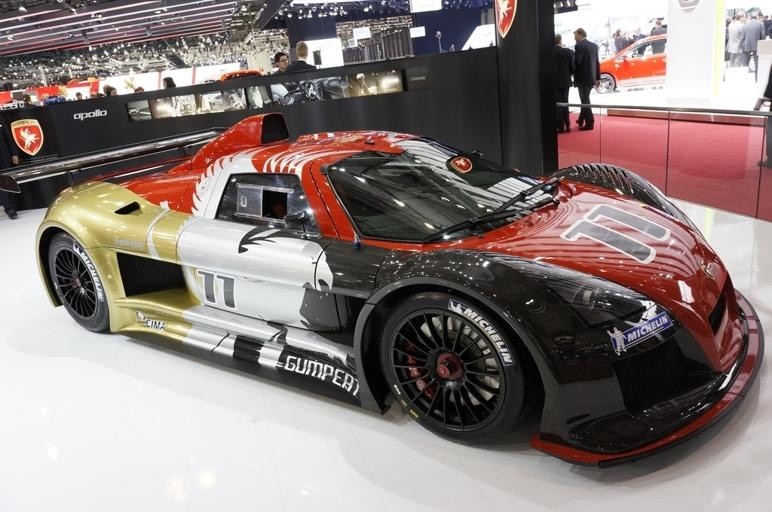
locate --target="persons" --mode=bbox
[573,27,602,130]
[285,41,316,76]
[725,13,771,75]
[161,76,177,90]
[756,61,772,169]
[272,52,292,75]
[22,85,119,107]
[612,16,666,58]
[554,33,576,133]
[133,86,145,93]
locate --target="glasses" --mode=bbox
[277,59,289,63]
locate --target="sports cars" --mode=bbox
[207,95,224,110]
[0,112,765,469]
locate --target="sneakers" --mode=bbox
[756,158,772,168]
[559,120,594,133]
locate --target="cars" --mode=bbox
[592,35,668,95]
